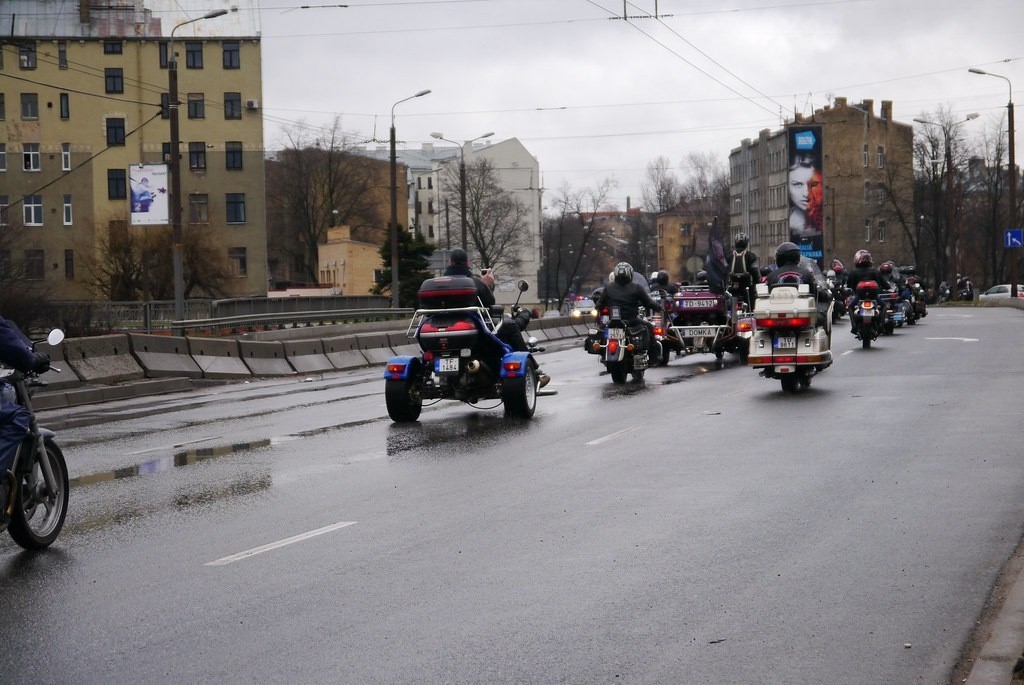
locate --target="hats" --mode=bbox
[450,249,468,264]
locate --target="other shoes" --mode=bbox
[537,375,550,387]
[922,311,928,317]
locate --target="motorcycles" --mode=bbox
[592,268,925,393]
[0,329,70,550]
[383,272,559,422]
[937,283,969,303]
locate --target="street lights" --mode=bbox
[969,68,1018,297]
[169,10,227,322]
[430,131,495,250]
[390,89,433,317]
[913,113,980,301]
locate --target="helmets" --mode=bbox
[834,265,844,273]
[854,250,872,267]
[906,266,916,275]
[734,233,749,248]
[880,264,891,275]
[615,262,633,280]
[776,242,800,267]
[657,272,669,283]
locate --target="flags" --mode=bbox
[707,217,730,298]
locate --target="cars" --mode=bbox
[570,297,596,316]
[541,310,563,318]
[979,283,1024,299]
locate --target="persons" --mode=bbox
[768,241,832,337]
[595,262,662,367]
[443,249,550,388]
[645,270,678,317]
[927,287,938,304]
[956,273,974,300]
[134,178,154,199]
[758,265,771,283]
[725,232,758,319]
[847,249,892,334]
[0,315,50,476]
[880,261,927,325]
[938,279,952,302]
[789,154,819,238]
[822,265,854,316]
[696,271,708,285]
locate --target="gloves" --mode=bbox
[33,351,51,374]
